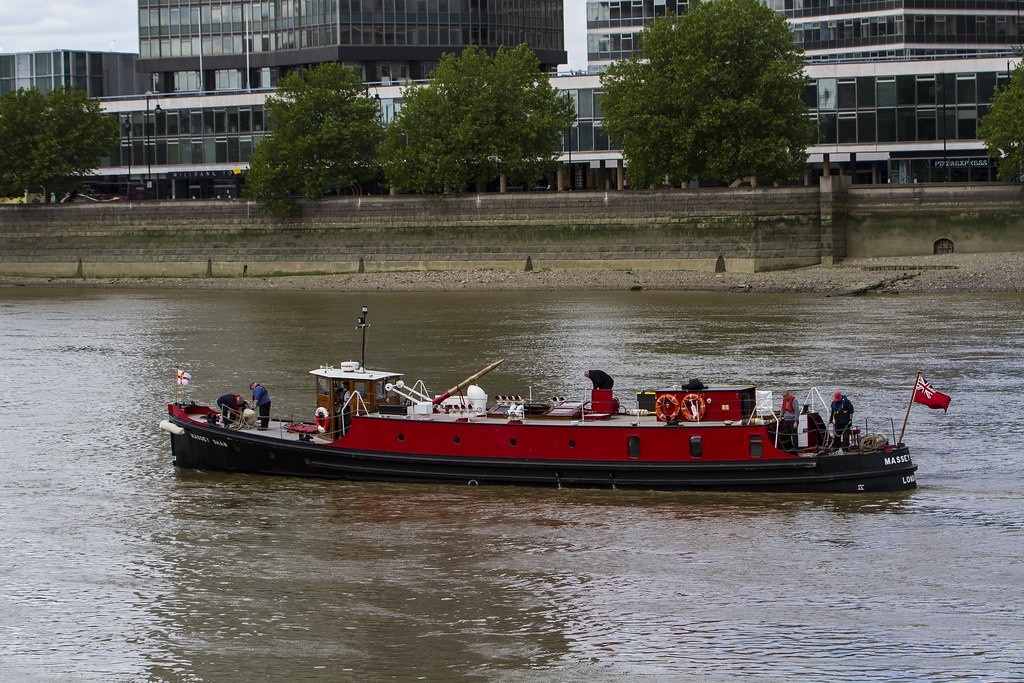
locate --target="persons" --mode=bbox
[780,388,800,450]
[829,391,854,450]
[248,382,271,430]
[342,380,351,433]
[216,393,245,424]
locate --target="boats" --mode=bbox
[160,305,919,492]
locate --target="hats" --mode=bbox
[249,382,255,390]
[833,392,841,401]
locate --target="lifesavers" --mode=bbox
[655,394,679,420]
[681,393,706,421]
[315,407,329,433]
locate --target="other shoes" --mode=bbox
[257,427,267,431]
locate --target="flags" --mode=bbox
[176,370,191,386]
[913,375,952,414]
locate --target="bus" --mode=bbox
[74,180,146,200]
[0,184,45,203]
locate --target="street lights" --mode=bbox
[124,112,131,179]
[366,84,381,102]
[146,96,162,180]
